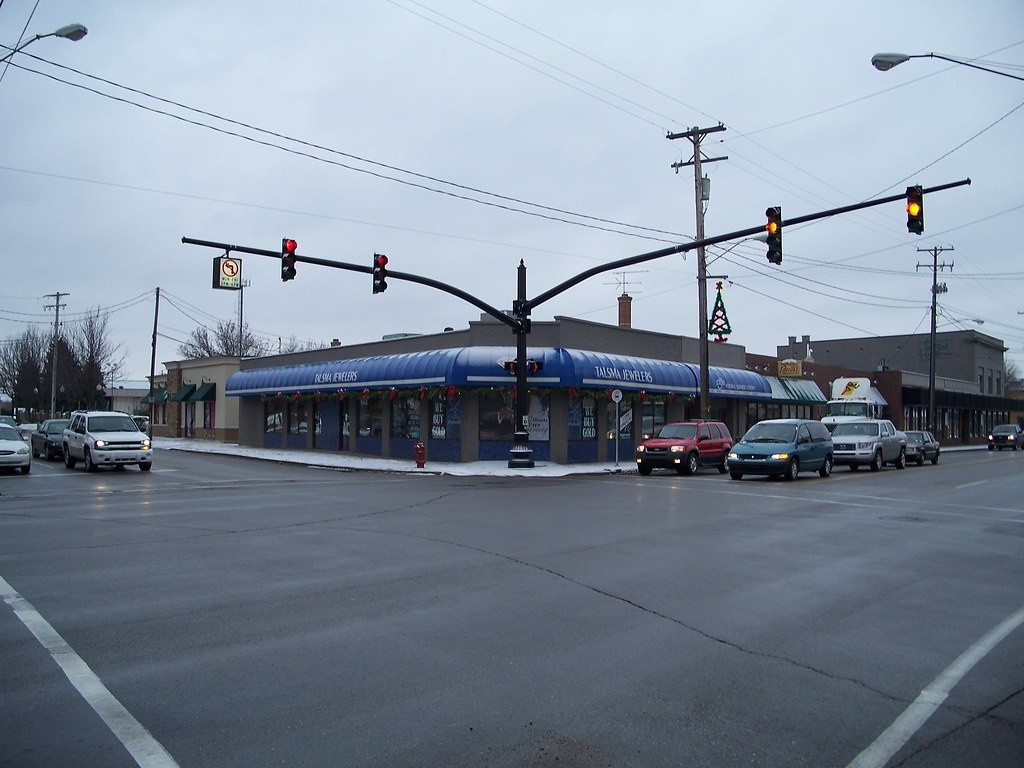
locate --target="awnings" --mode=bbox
[684,363,772,401]
[226,347,696,397]
[140,388,168,404]
[189,383,216,402]
[748,375,828,405]
[170,384,195,402]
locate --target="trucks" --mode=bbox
[820,397,883,436]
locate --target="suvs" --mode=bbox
[60,409,153,472]
[0,415,22,437]
[635,419,738,476]
[987,424,1024,451]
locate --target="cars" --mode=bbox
[728,419,835,482]
[897,431,941,467]
[830,420,908,472]
[0,424,31,474]
[30,419,72,462]
[133,416,150,432]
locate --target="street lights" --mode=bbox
[106,362,118,411]
[928,317,984,436]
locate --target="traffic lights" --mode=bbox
[281,239,298,282]
[526,360,544,374]
[905,185,925,235]
[765,206,784,264]
[372,253,389,295]
[504,361,516,372]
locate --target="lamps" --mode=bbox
[182,377,191,386]
[201,376,210,385]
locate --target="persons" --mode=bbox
[83,419,96,431]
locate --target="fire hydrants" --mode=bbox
[415,440,426,468]
